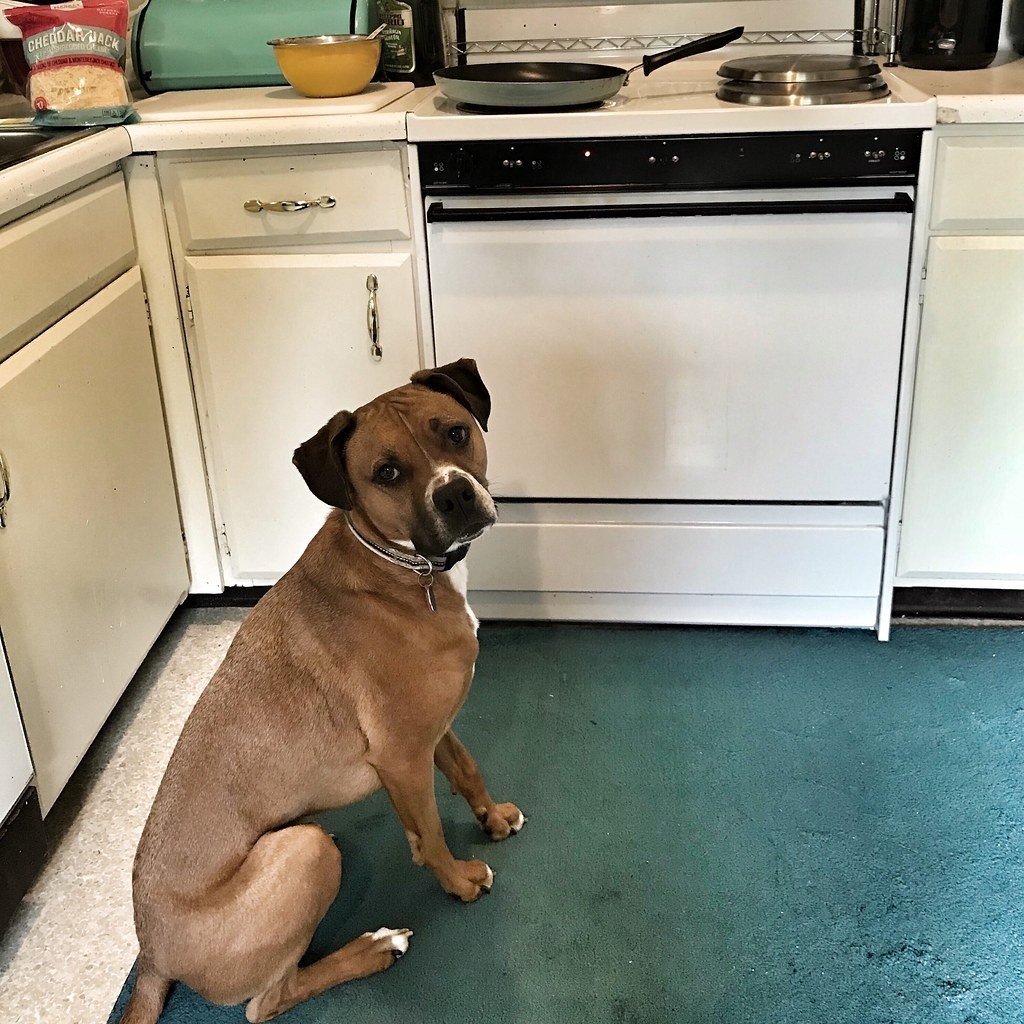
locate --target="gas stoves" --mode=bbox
[409,54,929,192]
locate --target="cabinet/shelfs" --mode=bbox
[0,169,191,821]
[875,124,1023,643]
[120,140,439,593]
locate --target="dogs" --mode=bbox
[115,355,530,1024]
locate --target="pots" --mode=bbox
[431,25,746,107]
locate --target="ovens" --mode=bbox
[422,193,916,627]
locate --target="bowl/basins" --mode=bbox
[266,34,386,98]
